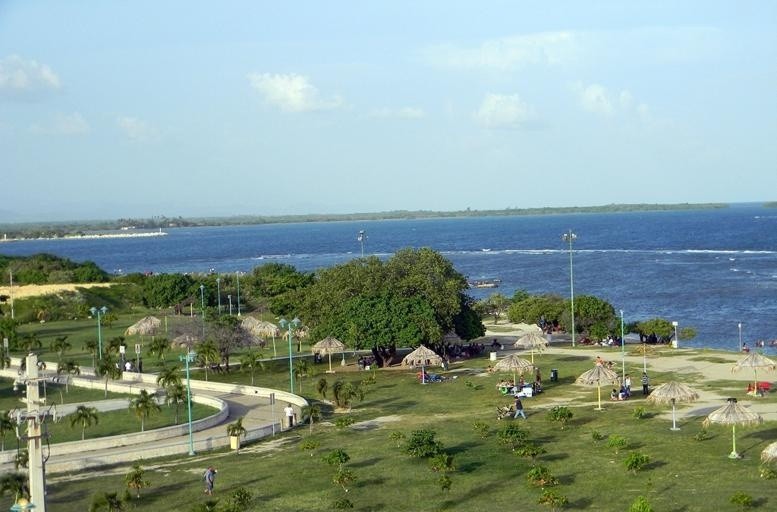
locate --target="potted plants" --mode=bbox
[227,415,248,451]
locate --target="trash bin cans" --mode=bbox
[551,369,558,381]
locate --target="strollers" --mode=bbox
[497,405,516,419]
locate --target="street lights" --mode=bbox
[179,352,198,456]
[279,317,301,394]
[563,228,577,347]
[90,305,109,360]
[200,270,241,318]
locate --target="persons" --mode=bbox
[513,395,527,420]
[741,339,776,352]
[593,355,603,365]
[419,370,430,383]
[607,360,611,369]
[620,385,630,397]
[115,356,144,374]
[357,354,376,370]
[537,315,625,348]
[617,389,628,400]
[624,373,631,397]
[416,338,503,361]
[639,371,649,395]
[316,352,322,363]
[203,465,216,496]
[609,388,617,400]
[496,368,541,395]
[427,373,446,382]
[283,402,294,427]
